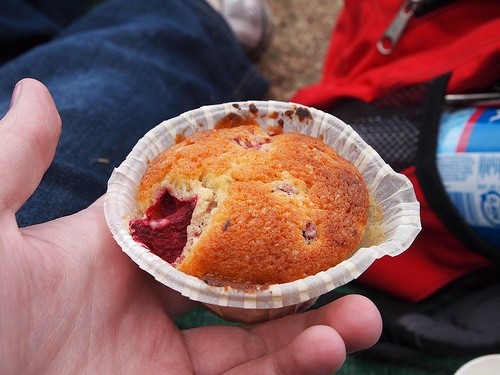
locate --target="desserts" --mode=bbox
[103,101,422,325]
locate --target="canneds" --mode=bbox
[352,101,500,246]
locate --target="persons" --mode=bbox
[0,0,383,375]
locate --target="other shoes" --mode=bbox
[205,0,275,58]
